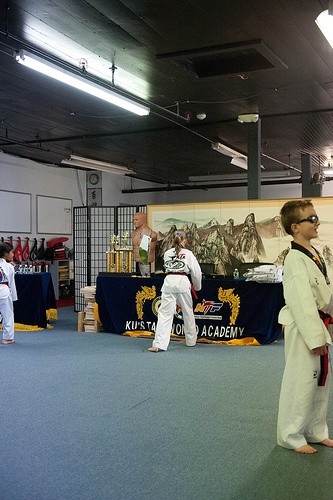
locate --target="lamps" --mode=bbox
[12,49,150,116]
[61,152,134,175]
[212,142,248,162]
[237,114,259,123]
[315,0,333,49]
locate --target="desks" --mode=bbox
[95,274,286,345]
[13,272,57,329]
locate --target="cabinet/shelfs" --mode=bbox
[48,260,70,301]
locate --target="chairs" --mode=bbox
[239,263,274,274]
[199,263,215,274]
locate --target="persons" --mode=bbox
[277,201,333,453]
[0,242,18,344]
[148,232,202,352]
[131,212,157,262]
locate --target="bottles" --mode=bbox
[18,265,24,274]
[23,265,28,274]
[234,269,239,280]
[28,265,33,274]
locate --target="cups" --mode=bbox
[139,264,150,276]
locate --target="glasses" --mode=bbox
[295,215,319,224]
